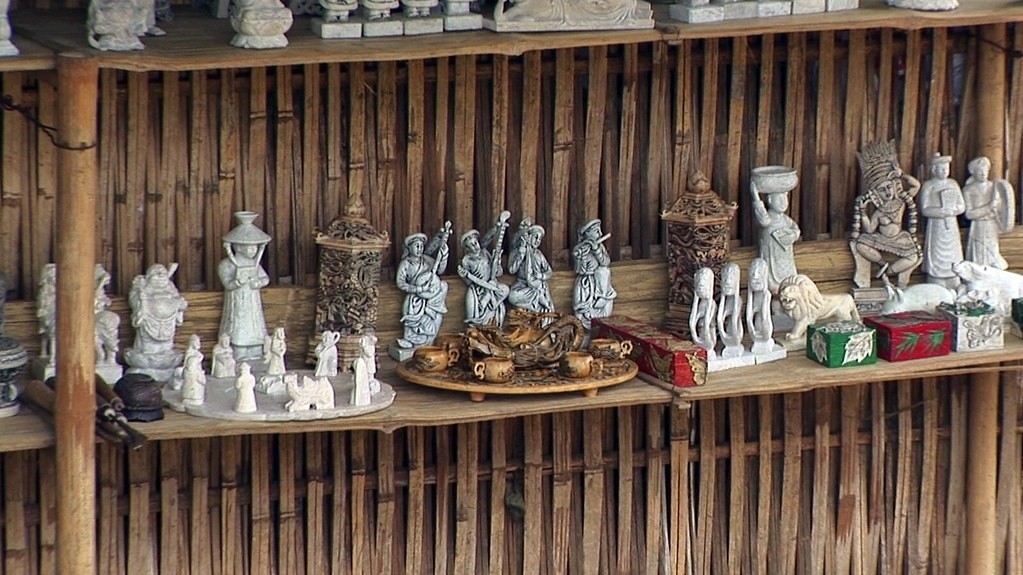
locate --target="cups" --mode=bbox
[412,330,634,382]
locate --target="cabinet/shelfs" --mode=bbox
[1,0,1023,573]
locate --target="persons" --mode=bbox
[750,181,801,287]
[217,224,271,362]
[457,218,555,328]
[182,327,377,415]
[855,155,1009,288]
[396,227,449,345]
[571,219,617,331]
[128,263,188,354]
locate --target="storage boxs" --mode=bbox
[937,302,1005,353]
[862,309,951,363]
[807,320,876,369]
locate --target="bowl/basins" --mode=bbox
[112,373,165,423]
